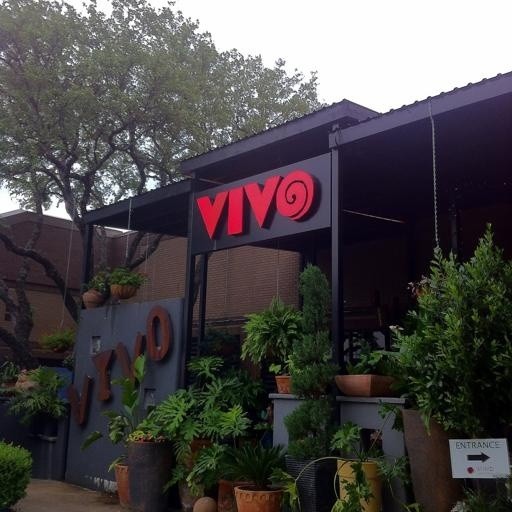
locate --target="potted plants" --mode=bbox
[109,328,240,510]
[82,270,110,310]
[109,267,147,300]
[240,224,512,511]
